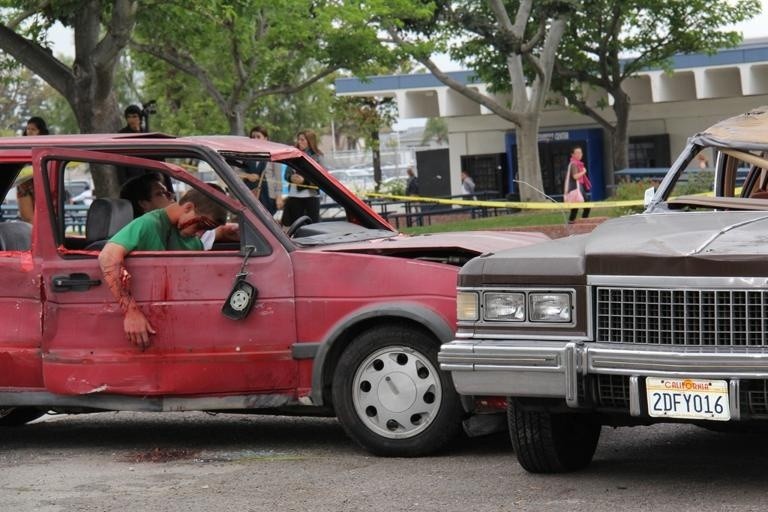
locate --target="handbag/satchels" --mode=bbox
[563,189,584,203]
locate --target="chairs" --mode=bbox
[0,198,136,252]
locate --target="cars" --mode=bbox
[0,133,551,456]
[435,105,768,474]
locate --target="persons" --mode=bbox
[14,116,49,225]
[98,183,228,349]
[119,170,240,250]
[404,168,422,214]
[460,168,476,197]
[285,129,326,235]
[118,105,151,134]
[236,127,283,219]
[698,152,708,169]
[568,147,592,222]
